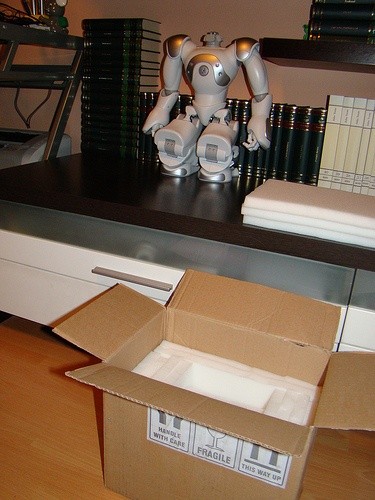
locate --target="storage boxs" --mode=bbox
[52,268,375,500]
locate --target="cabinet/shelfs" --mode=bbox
[0,154,375,352]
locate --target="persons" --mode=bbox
[143,31,272,183]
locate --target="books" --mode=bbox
[317,95,375,196]
[138,91,327,192]
[310,0,375,41]
[241,177,375,251]
[80,17,162,160]
